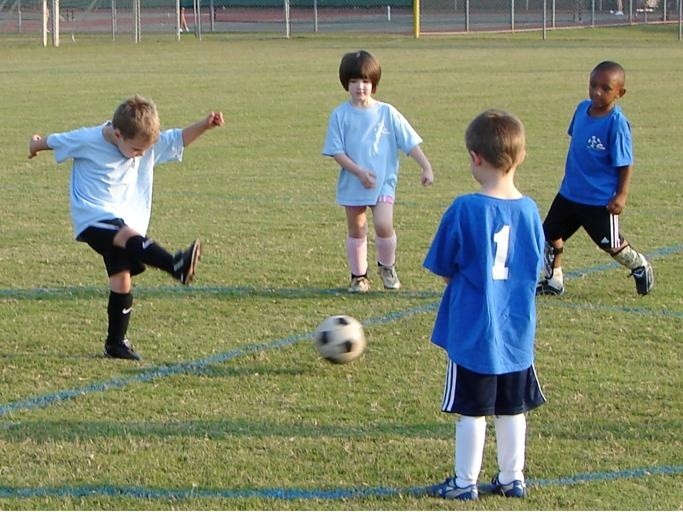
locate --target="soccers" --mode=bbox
[316,315,366,363]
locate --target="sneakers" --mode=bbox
[105,334,140,360]
[627,253,654,295]
[347,274,369,294]
[477,471,524,498]
[536,277,565,296]
[424,478,478,500]
[376,262,401,290]
[171,239,201,286]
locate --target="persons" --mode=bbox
[421,106,547,501]
[26,92,225,361]
[614,0,624,17]
[531,58,656,295]
[179,7,190,34]
[321,47,437,292]
